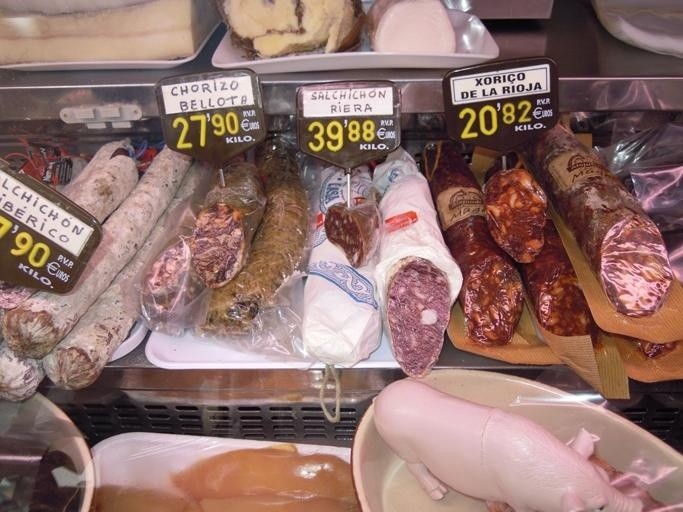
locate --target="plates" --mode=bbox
[349,371,682,511]
[0,390,97,512]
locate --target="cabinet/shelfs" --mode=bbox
[1,1,683,444]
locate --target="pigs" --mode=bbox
[368,376,644,512]
